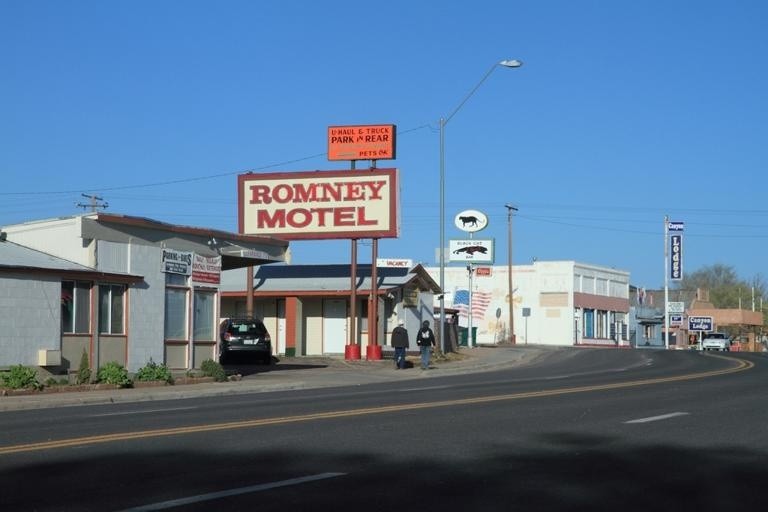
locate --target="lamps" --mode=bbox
[207,235,217,246]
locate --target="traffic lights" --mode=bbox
[688,333,698,345]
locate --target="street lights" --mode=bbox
[440,59,522,354]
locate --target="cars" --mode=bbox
[219,319,270,365]
[703,333,729,352]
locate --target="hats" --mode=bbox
[397,318,405,325]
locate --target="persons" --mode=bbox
[391,319,410,370]
[414,319,436,369]
